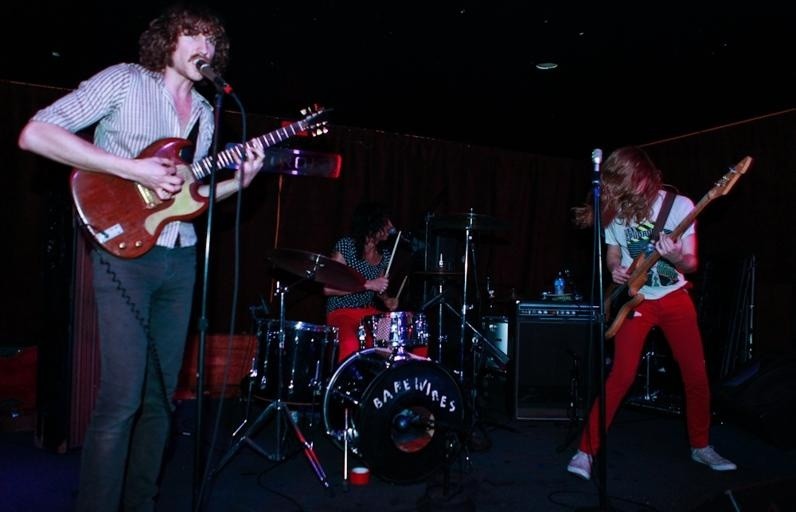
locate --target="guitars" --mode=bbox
[604,156,753,341]
[68,103,329,260]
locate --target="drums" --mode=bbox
[476,316,511,380]
[249,318,340,406]
[323,347,466,486]
[364,310,431,347]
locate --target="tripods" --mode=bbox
[418,232,511,449]
[211,286,332,487]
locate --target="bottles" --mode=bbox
[555,270,576,295]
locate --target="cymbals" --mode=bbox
[264,248,366,292]
[413,271,463,281]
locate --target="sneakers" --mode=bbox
[566,449,594,481]
[690,444,739,472]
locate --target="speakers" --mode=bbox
[514,318,607,421]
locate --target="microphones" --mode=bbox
[590,147,603,187]
[195,57,231,91]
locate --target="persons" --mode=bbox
[321,201,431,486]
[17,11,266,512]
[567,144,738,481]
